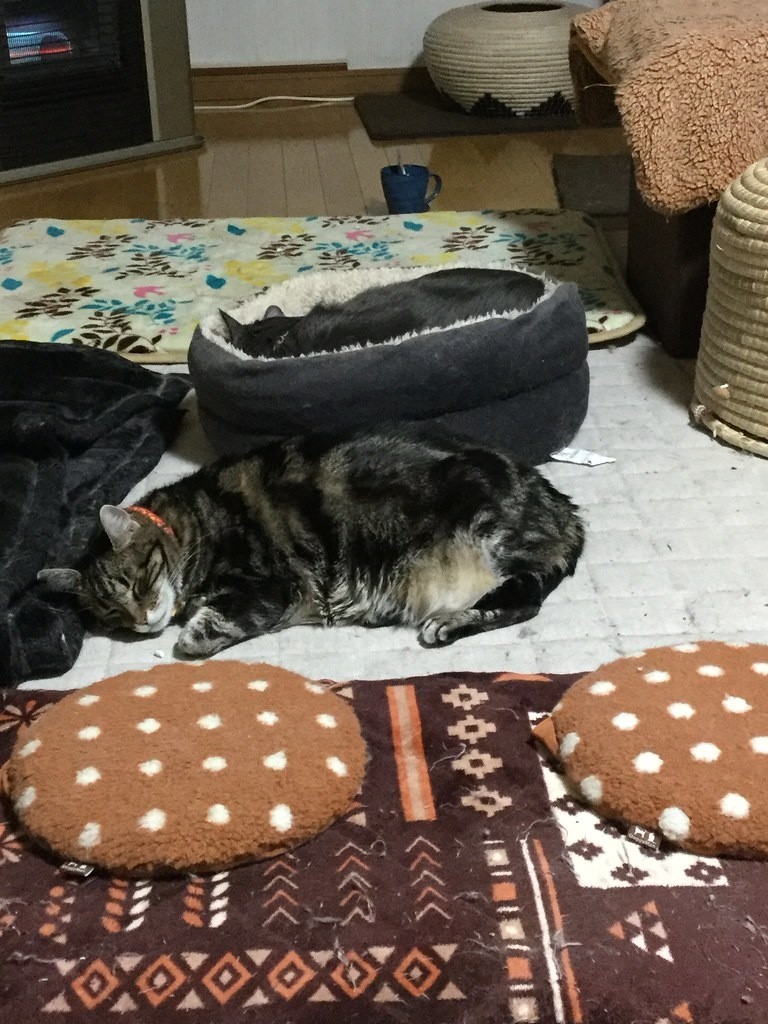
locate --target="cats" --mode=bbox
[217,267,545,359]
[36,432,586,659]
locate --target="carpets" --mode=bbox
[352,90,620,143]
[0,208,649,365]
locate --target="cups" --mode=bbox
[380,164,443,214]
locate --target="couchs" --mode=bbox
[569,0,768,358]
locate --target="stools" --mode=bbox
[423,0,596,118]
